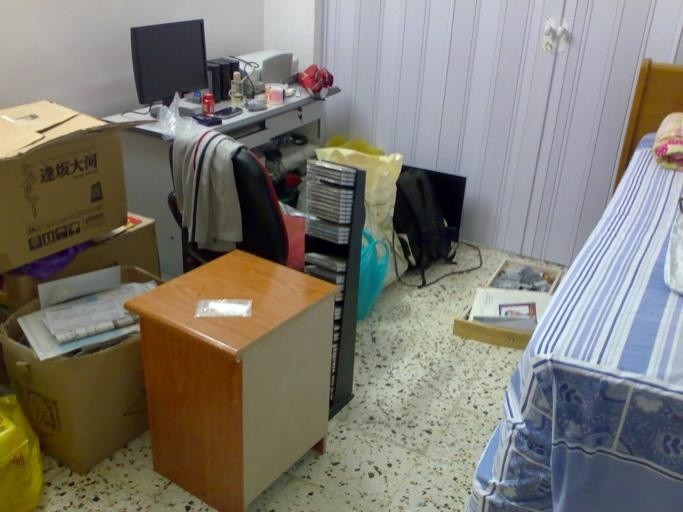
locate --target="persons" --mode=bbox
[505,311,528,316]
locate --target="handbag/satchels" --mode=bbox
[296,65,334,102]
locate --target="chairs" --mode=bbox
[164,123,312,273]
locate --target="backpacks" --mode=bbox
[394,170,458,287]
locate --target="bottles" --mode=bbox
[192,87,201,103]
[202,93,215,119]
[229,71,244,111]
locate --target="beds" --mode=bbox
[466,51,682,512]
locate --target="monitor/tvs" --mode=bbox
[393,165,465,242]
[131,18,209,117]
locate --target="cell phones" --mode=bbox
[192,114,222,126]
[213,107,241,119]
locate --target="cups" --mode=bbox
[264,83,283,106]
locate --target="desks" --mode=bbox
[121,245,344,512]
[101,82,347,285]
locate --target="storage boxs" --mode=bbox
[1,209,168,314]
[0,97,130,277]
[1,261,167,480]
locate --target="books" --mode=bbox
[466,281,552,333]
[303,159,356,403]
